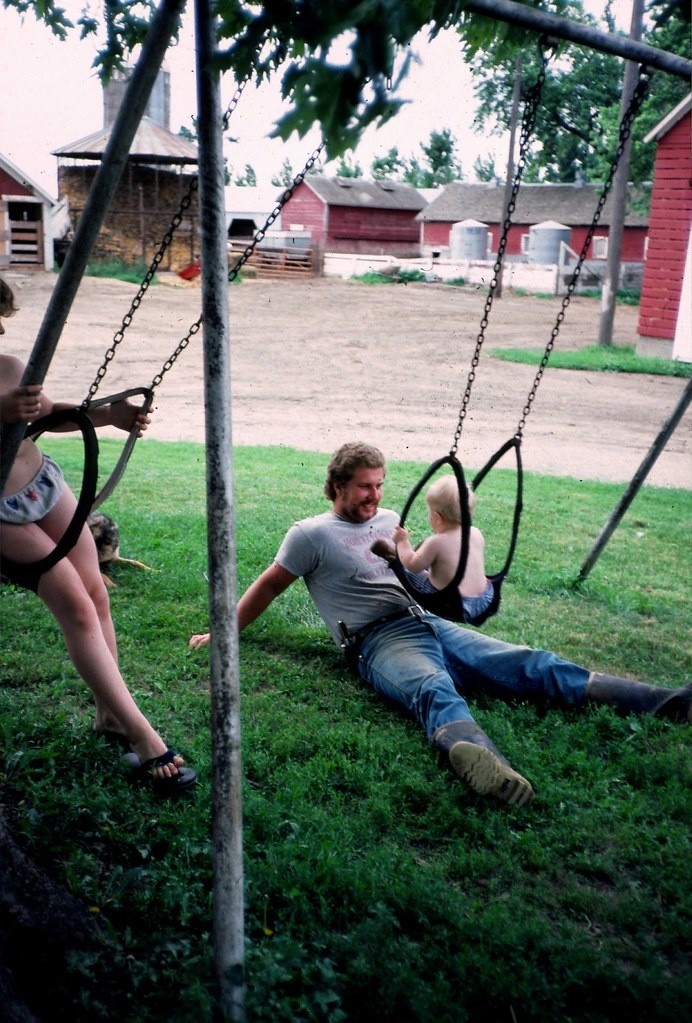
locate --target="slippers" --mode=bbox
[122,749,198,790]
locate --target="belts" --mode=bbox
[353,605,430,639]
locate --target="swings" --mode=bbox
[387,42,659,629]
[0,1,384,589]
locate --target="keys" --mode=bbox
[337,619,351,640]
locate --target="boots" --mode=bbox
[432,720,534,809]
[588,669,692,720]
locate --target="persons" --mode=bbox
[189,442,692,808]
[369,474,494,619]
[0,281,198,786]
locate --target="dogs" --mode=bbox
[87,510,163,588]
